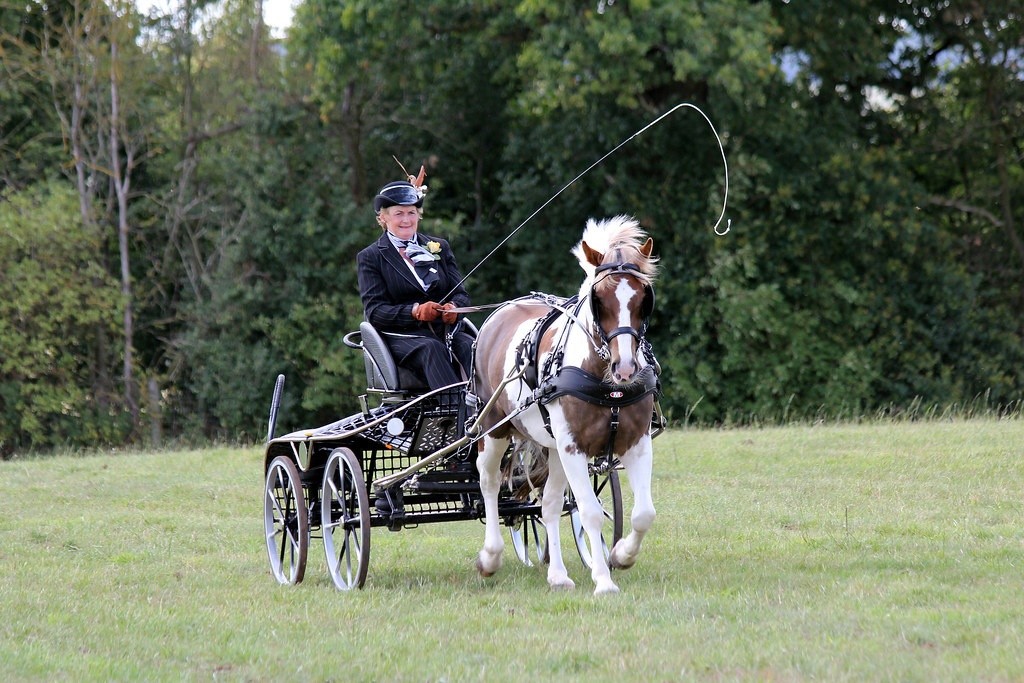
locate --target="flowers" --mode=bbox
[423,241,443,254]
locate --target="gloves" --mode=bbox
[411,301,444,321]
[442,302,458,324]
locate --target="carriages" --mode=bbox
[263,214,667,598]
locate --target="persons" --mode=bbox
[356,181,525,475]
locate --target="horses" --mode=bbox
[468,215,663,596]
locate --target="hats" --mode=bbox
[373,155,428,216]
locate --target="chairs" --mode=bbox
[344,317,479,396]
